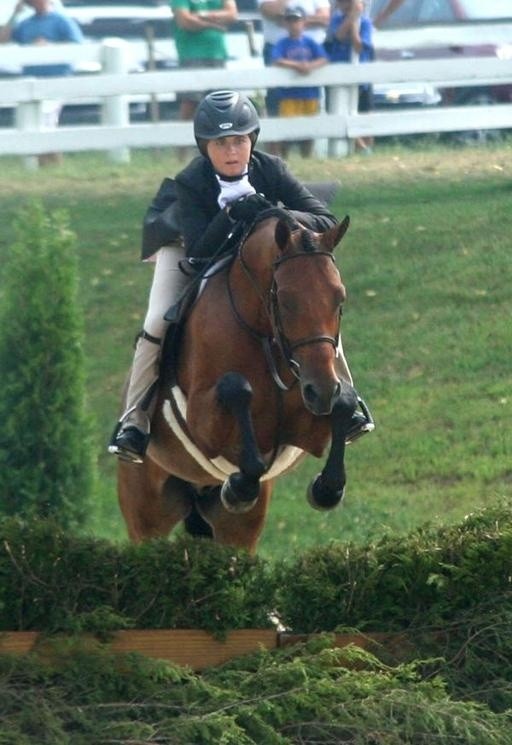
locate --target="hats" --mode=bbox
[284,6,306,20]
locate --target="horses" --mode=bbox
[115,214,358,558]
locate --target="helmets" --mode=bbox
[194,91,260,139]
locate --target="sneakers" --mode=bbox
[346,412,367,433]
[114,425,144,454]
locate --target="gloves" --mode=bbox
[226,192,273,224]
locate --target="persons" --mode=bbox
[0,0,86,169]
[325,0,372,155]
[269,6,329,160]
[115,93,369,459]
[169,0,239,164]
[258,0,331,157]
